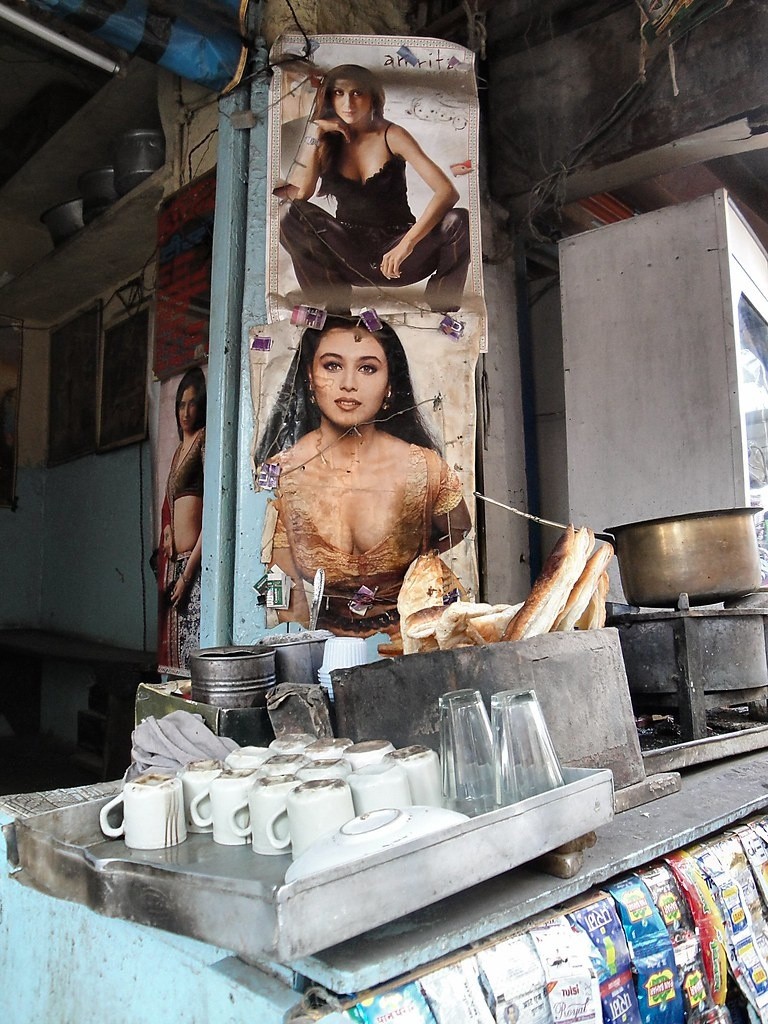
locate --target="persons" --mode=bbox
[278,63,470,317]
[149,366,207,681]
[254,313,472,636]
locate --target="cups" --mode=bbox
[318,637,368,703]
[100,733,440,861]
[438,689,567,819]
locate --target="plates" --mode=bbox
[285,804,475,883]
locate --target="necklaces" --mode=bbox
[182,436,193,452]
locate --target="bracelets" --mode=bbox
[303,137,320,148]
[181,574,194,583]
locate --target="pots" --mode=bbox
[472,490,763,609]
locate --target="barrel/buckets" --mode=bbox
[189,638,328,748]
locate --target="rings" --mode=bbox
[380,264,383,266]
